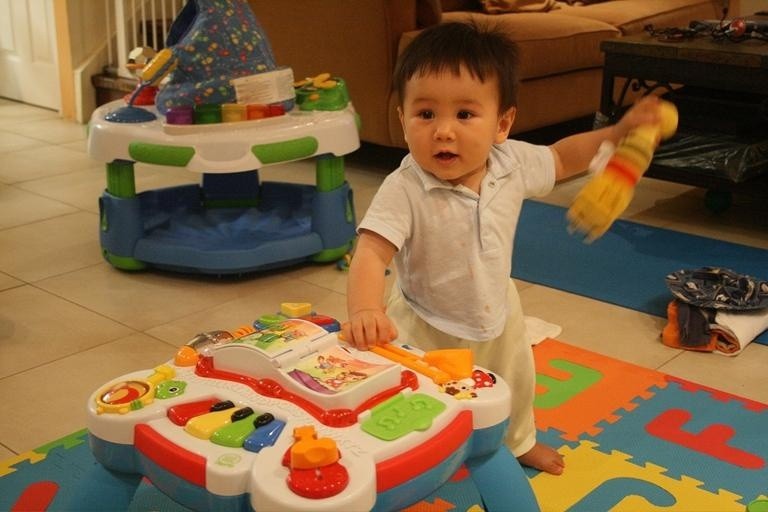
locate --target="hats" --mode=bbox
[665,266,768,311]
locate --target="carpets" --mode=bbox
[513,201,767,346]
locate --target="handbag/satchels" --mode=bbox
[155,0,281,116]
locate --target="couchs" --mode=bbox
[250,1,741,167]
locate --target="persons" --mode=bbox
[344,20,661,477]
